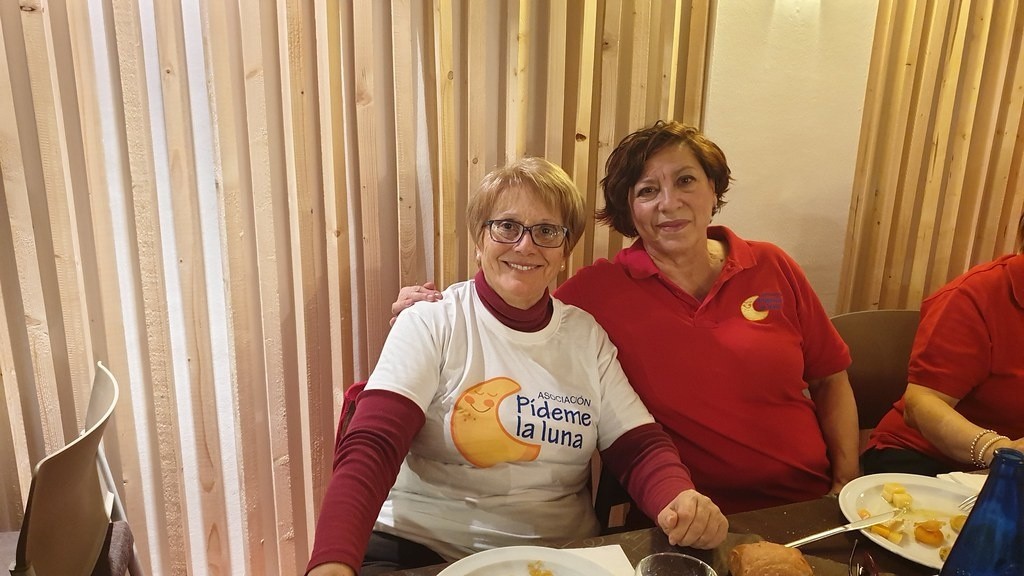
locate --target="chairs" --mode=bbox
[0,362,147,576]
[827,308,928,438]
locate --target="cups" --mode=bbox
[634,553,718,576]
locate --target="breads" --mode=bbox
[729,541,814,576]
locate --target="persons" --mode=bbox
[863,210,1024,480]
[389,118,861,522]
[297,156,731,576]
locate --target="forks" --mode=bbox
[958,494,978,511]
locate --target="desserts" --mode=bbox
[858,483,968,560]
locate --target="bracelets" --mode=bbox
[978,435,1011,468]
[970,429,1000,470]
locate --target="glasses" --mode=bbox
[482,219,568,248]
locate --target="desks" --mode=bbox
[365,466,991,576]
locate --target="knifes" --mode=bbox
[784,510,904,548]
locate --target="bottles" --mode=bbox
[939,448,1024,576]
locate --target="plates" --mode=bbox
[838,473,981,569]
[435,546,616,576]
